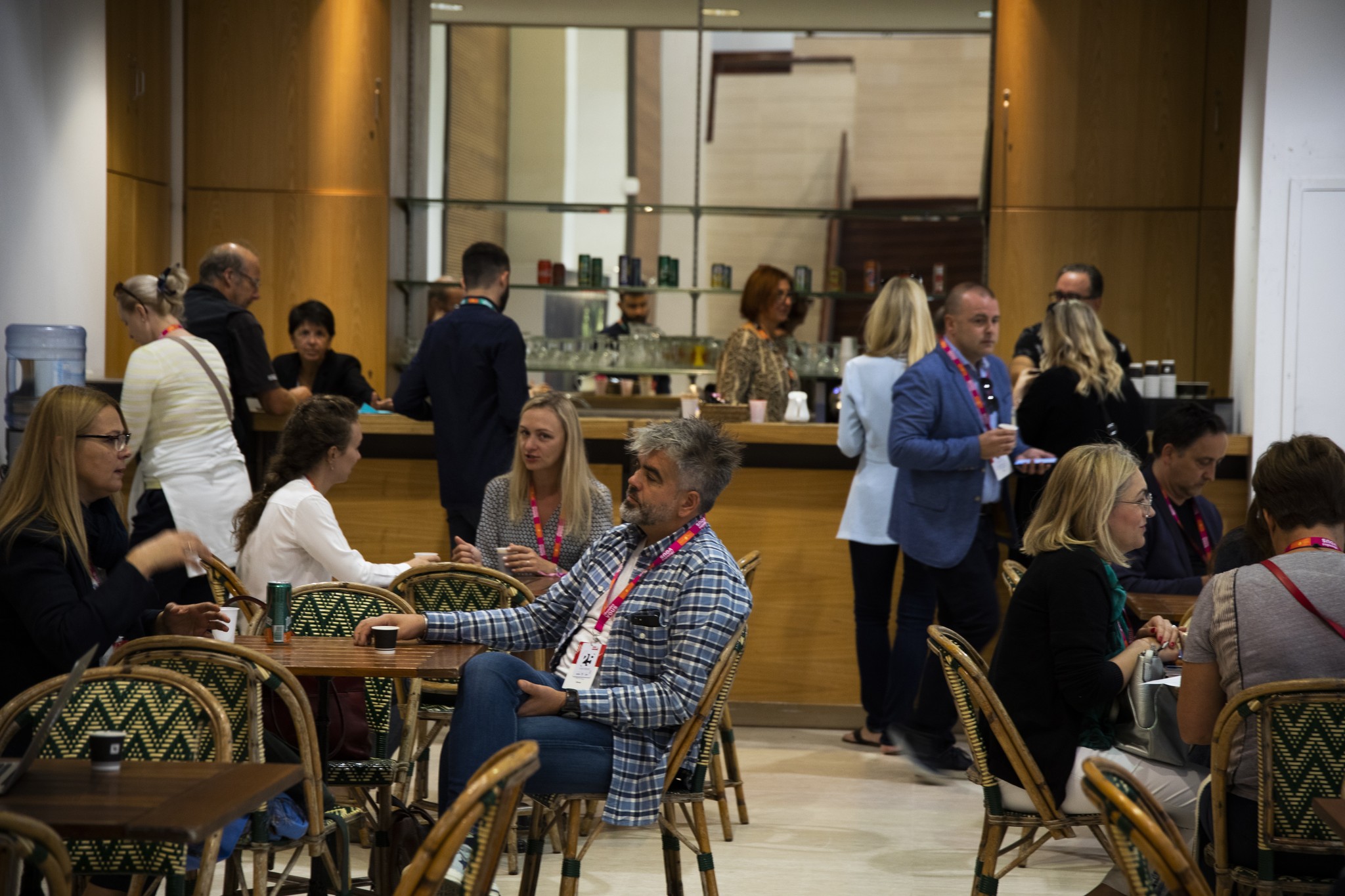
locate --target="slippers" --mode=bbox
[842,726,880,747]
[884,749,904,756]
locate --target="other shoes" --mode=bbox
[503,837,527,853]
[444,843,472,884]
[930,745,973,779]
[516,815,530,830]
[893,726,952,787]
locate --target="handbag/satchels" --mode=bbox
[1114,648,1195,767]
[369,795,436,896]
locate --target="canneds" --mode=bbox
[670,259,678,287]
[864,260,877,293]
[631,258,641,286]
[711,263,725,288]
[578,253,593,287]
[657,255,672,287]
[265,580,292,646]
[592,258,603,287]
[934,263,946,294]
[553,263,565,286]
[725,266,731,289]
[806,269,812,291]
[794,265,807,291]
[538,259,553,285]
[827,266,843,293]
[619,255,634,286]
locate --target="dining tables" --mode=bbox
[370,572,565,873]
[170,634,488,896]
[0,754,306,894]
[1123,591,1200,621]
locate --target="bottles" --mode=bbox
[4,321,86,431]
[526,333,841,376]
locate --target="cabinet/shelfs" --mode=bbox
[384,194,990,423]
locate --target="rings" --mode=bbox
[1171,625,1177,627]
[187,539,193,553]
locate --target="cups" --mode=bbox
[494,546,519,578]
[750,399,768,424]
[88,730,125,773]
[414,551,436,558]
[997,423,1018,432]
[213,606,239,644]
[680,397,700,419]
[370,625,399,656]
[1129,361,1178,398]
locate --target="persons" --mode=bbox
[1105,400,1228,597]
[588,279,674,395]
[777,298,816,416]
[396,239,529,553]
[888,279,1060,782]
[271,297,396,411]
[0,386,232,896]
[349,415,754,895]
[428,276,465,324]
[1005,261,1133,390]
[233,393,442,638]
[714,264,803,425]
[1177,432,1345,896]
[182,241,315,479]
[833,276,935,748]
[1010,295,1158,569]
[977,438,1206,896]
[111,260,253,611]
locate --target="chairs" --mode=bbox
[223,581,426,896]
[1079,752,1217,896]
[1212,678,1345,896]
[567,546,764,842]
[1002,560,1131,870]
[195,546,266,634]
[394,737,544,896]
[925,624,1171,896]
[2,660,232,896]
[106,631,361,896]
[376,560,565,854]
[520,621,750,896]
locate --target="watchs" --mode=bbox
[556,690,581,719]
[554,564,563,575]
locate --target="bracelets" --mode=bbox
[1141,639,1160,656]
[421,614,429,640]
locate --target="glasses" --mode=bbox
[76,431,132,450]
[114,282,149,315]
[1047,290,1093,303]
[1115,493,1153,518]
[235,271,259,288]
